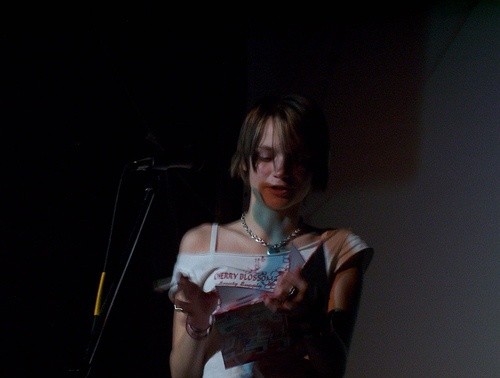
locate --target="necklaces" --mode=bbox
[241,211,306,255]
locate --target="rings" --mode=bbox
[287,286,297,300]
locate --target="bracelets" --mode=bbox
[185,315,213,339]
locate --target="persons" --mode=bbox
[168,95,374,378]
